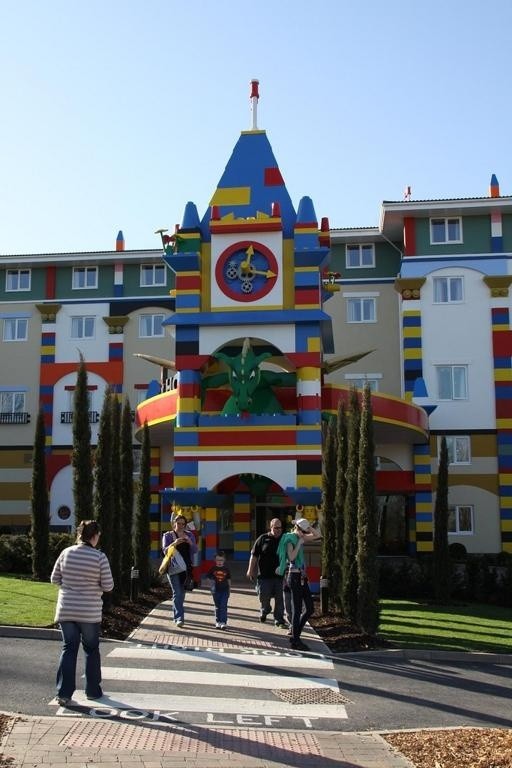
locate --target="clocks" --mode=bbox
[215,241,278,302]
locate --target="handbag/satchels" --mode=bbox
[159,544,175,575]
[167,545,187,576]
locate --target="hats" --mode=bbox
[296,518,311,535]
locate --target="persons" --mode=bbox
[48,520,114,705]
[158,515,323,652]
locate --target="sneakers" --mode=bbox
[287,628,293,635]
[221,623,227,629]
[176,620,184,627]
[87,693,103,700]
[215,622,220,628]
[260,610,267,623]
[56,697,69,706]
[289,636,310,651]
[274,623,288,629]
